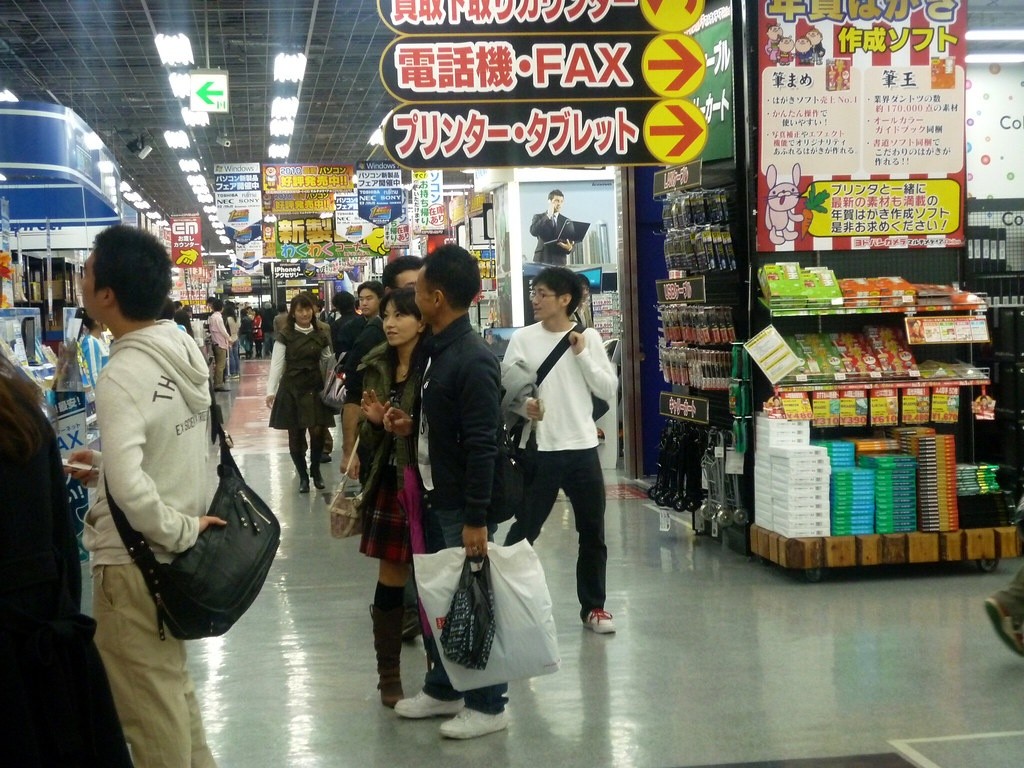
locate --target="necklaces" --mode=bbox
[398,365,409,378]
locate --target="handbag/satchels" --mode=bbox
[328,488,364,539]
[490,426,524,524]
[155,465,281,640]
[321,351,348,409]
[413,539,561,692]
[327,311,335,324]
[1,591,135,768]
[319,344,332,388]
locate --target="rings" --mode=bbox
[471,546,478,553]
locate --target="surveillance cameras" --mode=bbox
[137,146,153,159]
[216,136,230,147]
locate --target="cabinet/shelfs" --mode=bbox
[747,285,1020,584]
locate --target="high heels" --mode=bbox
[298,469,309,492]
[310,466,325,489]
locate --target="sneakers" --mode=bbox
[440,706,509,739]
[583,608,616,634]
[394,689,465,718]
[984,596,1024,656]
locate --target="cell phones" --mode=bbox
[61,458,92,470]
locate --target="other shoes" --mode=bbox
[320,453,332,463]
[227,373,240,378]
[214,387,231,392]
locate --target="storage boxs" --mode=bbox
[753,414,959,539]
[778,325,919,384]
[757,265,917,308]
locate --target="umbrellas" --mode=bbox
[404,437,432,676]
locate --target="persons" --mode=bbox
[984,560,1024,664]
[64,223,227,768]
[73,306,109,427]
[160,296,262,392]
[0,353,135,768]
[571,272,610,439]
[529,189,574,265]
[332,244,510,739]
[263,289,359,493]
[501,267,623,633]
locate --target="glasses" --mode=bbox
[359,296,373,302]
[530,292,556,301]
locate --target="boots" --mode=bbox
[369,604,405,707]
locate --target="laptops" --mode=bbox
[544,220,591,250]
[575,267,603,297]
